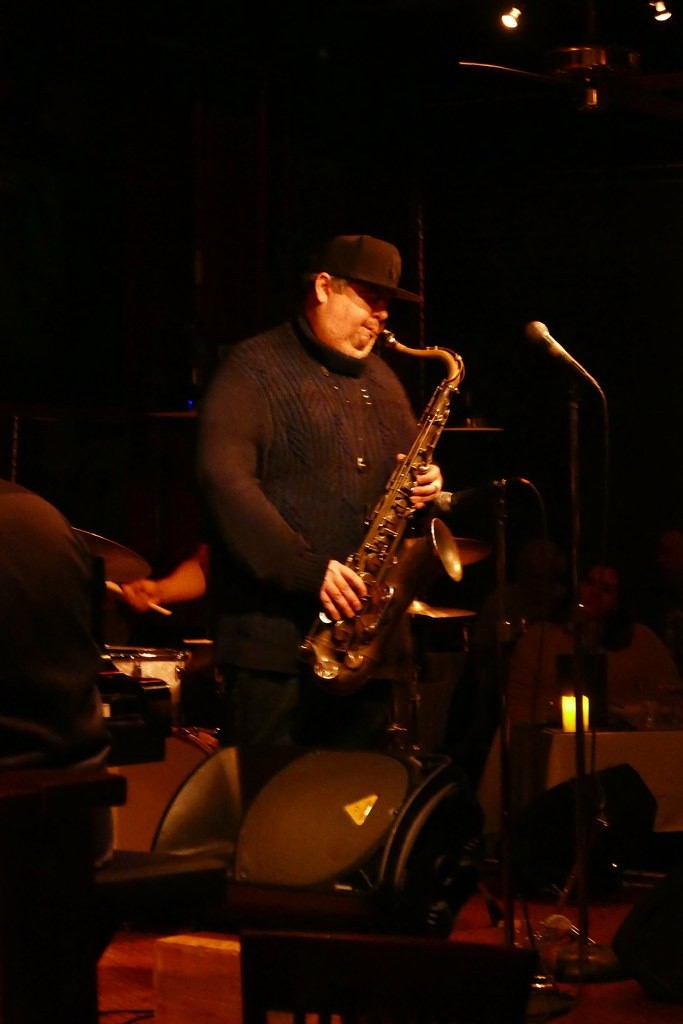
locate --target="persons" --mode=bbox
[198,233,443,824]
[507,551,680,732]
[123,542,211,613]
[0,476,109,1022]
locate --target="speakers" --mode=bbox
[612,862,683,1005]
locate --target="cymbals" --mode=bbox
[429,536,489,568]
[69,525,152,587]
[404,597,477,619]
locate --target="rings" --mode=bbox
[432,481,442,492]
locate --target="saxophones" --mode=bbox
[297,325,467,696]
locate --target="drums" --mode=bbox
[105,643,189,724]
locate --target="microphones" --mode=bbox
[434,479,513,512]
[526,321,601,390]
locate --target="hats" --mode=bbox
[311,235,425,304]
